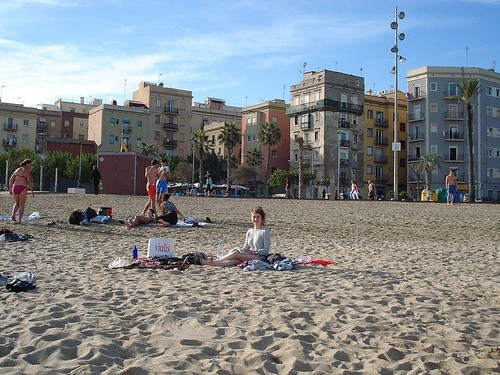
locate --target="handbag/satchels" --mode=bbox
[148,238,175,258]
[100,206,113,218]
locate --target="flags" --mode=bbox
[122,143,128,152]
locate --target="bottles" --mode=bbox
[133,245,138,259]
[218,242,223,259]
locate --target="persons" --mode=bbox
[349,179,360,200]
[90,165,102,196]
[203,171,213,197]
[200,205,271,267]
[9,159,35,224]
[420,188,468,203]
[368,179,377,200]
[445,170,457,206]
[124,159,186,228]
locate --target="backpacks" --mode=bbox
[69,210,83,225]
[83,208,98,224]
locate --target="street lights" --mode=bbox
[388,5,407,201]
